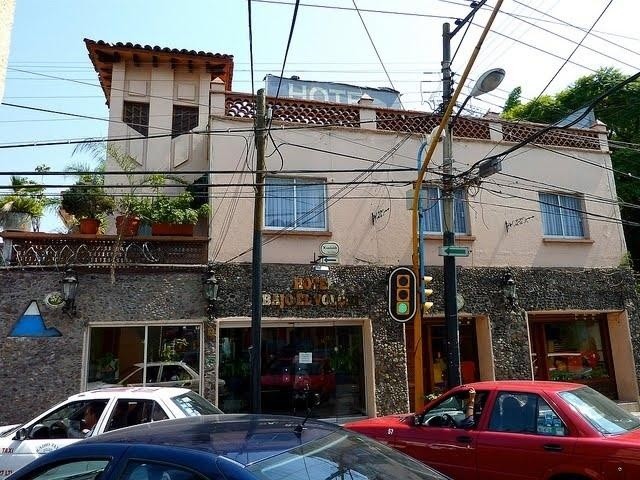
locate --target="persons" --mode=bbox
[502,396,522,424]
[463,387,485,429]
[58,403,104,439]
[114,402,129,417]
[432,350,475,391]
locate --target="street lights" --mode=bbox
[441,65,508,388]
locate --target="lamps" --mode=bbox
[199,256,221,319]
[499,263,522,316]
[58,259,83,319]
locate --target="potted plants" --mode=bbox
[0,187,45,234]
[60,181,117,235]
[111,192,144,236]
[138,173,212,239]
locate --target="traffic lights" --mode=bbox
[425,277,433,314]
[389,266,416,323]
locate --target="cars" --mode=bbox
[558,347,599,372]
[89,361,226,403]
[337,377,640,480]
[167,353,199,370]
[255,356,335,404]
[2,412,455,479]
[1,386,226,479]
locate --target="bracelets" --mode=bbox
[467,402,475,409]
